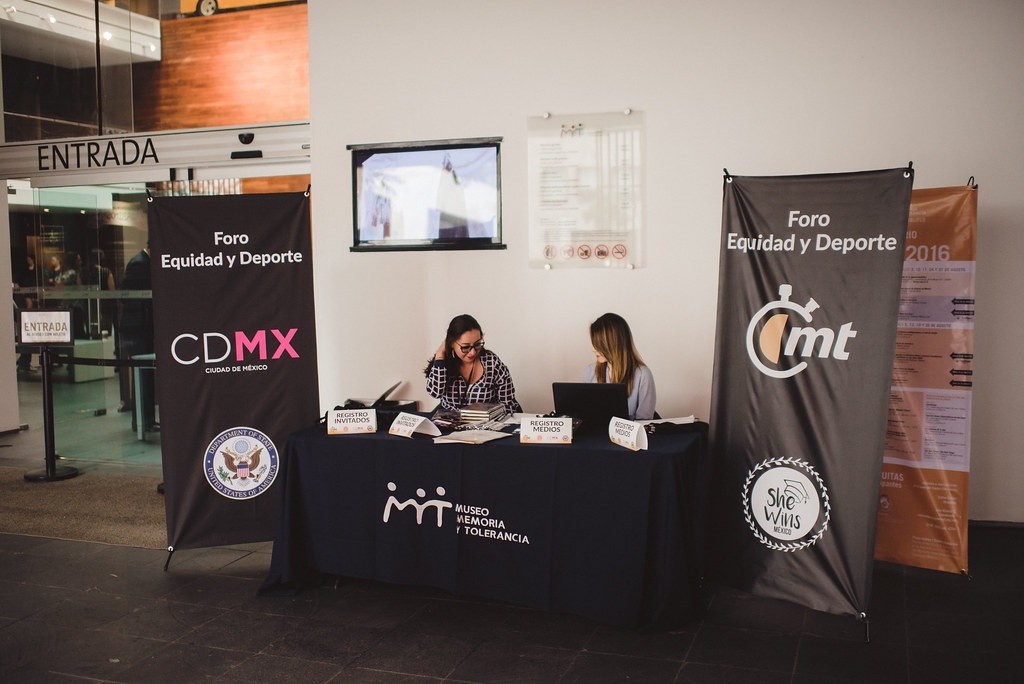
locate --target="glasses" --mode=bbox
[455,338,485,352]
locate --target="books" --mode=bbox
[460,403,506,423]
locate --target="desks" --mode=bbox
[132,353,156,440]
[258,422,709,628]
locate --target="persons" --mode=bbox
[425,314,519,414]
[13,238,116,373]
[590,313,656,420]
[117,238,160,431]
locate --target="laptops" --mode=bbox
[552,382,629,434]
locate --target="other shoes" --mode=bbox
[131,420,161,433]
